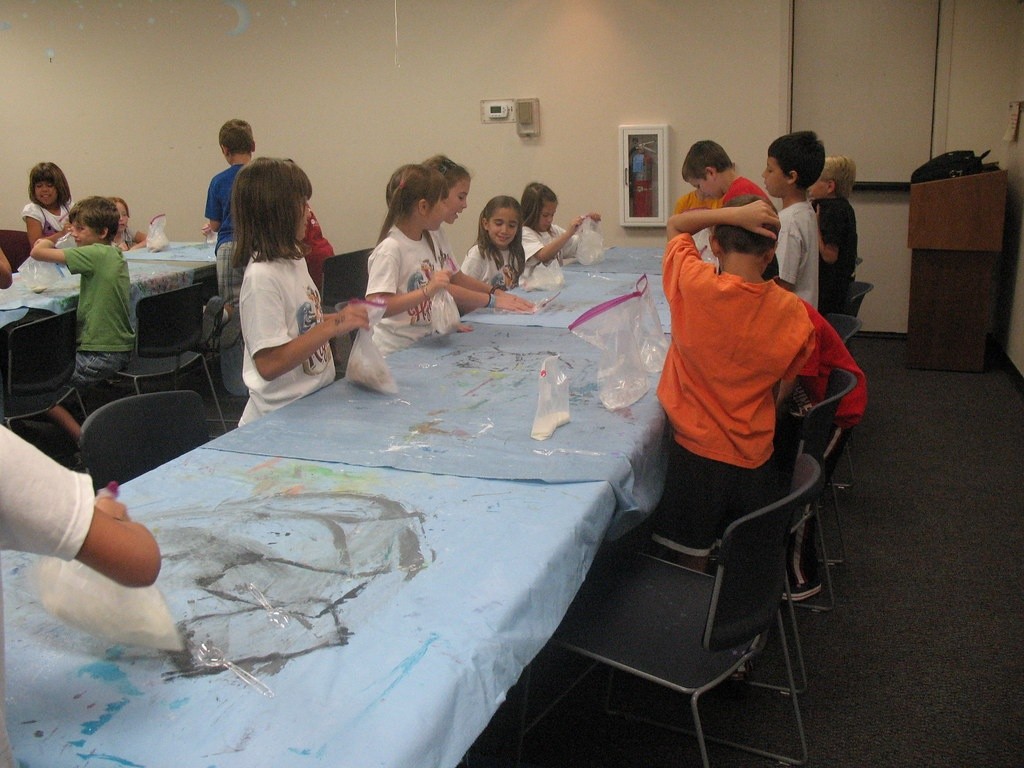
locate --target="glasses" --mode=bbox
[441,158,455,174]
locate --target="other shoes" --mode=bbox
[73,442,90,474]
[201,296,228,343]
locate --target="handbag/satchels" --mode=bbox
[911,150,991,183]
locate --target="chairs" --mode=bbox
[320,249,377,346]
[514,446,831,768]
[81,389,207,496]
[112,282,227,438]
[4,310,88,440]
[780,366,861,614]
[828,309,866,349]
[848,280,873,315]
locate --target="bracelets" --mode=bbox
[489,284,502,295]
[483,293,496,309]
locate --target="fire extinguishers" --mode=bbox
[629,140,656,218]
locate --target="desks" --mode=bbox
[0,241,715,768]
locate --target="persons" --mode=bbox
[22,163,75,248]
[655,129,867,603]
[0,421,161,588]
[200,118,256,300]
[28,194,150,444]
[228,157,370,430]
[0,244,13,289]
[364,155,601,362]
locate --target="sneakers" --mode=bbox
[782,579,822,601]
